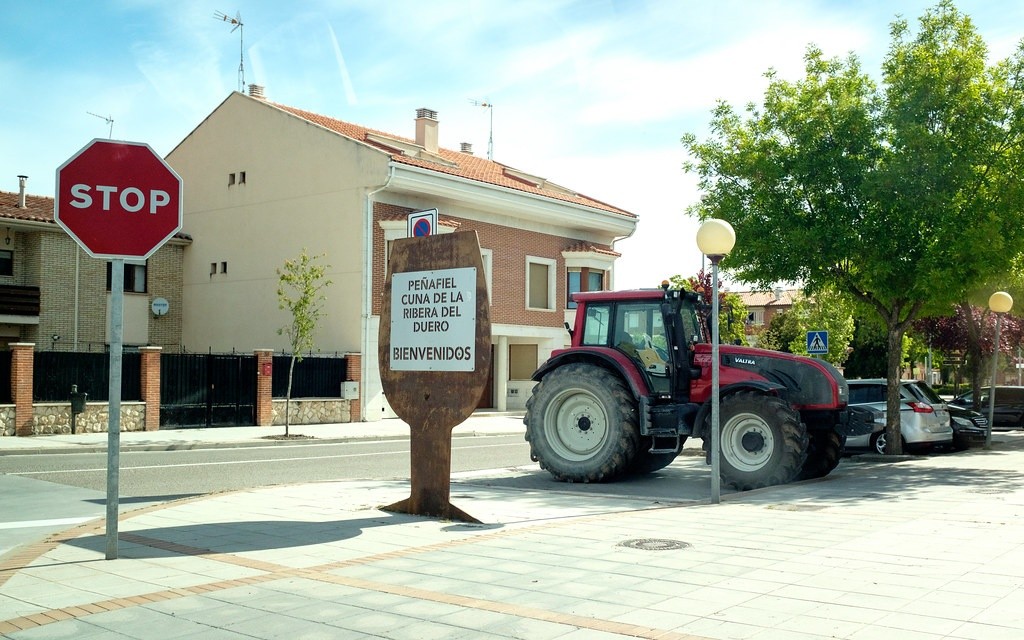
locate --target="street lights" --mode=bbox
[697,219,739,503]
[987,292,1014,437]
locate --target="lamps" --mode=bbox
[5,237,11,244]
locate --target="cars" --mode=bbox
[845,379,954,454]
[952,385,1024,428]
[944,401,989,446]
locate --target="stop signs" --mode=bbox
[53,139,183,262]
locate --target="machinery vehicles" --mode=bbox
[524,279,884,487]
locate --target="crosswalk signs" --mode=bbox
[806,330,828,356]
